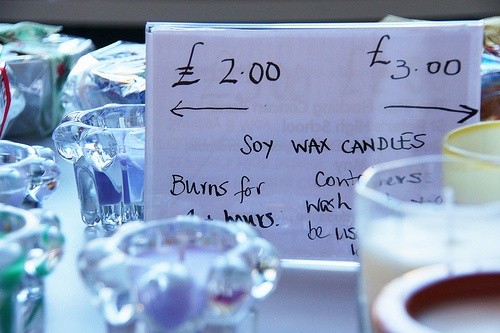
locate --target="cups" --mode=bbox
[1,202,65,333]
[0,140,60,211]
[75,216,282,333]
[345,155,500,333]
[440,122,498,205]
[46,105,146,233]
[371,258,500,333]
